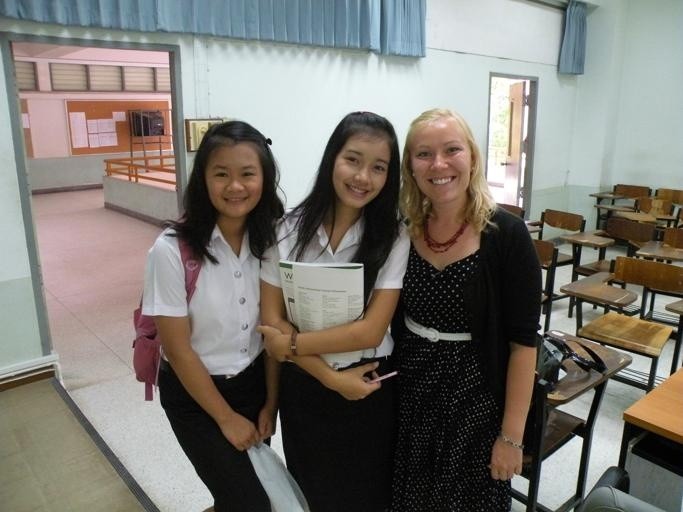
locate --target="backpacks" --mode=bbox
[131,222,203,400]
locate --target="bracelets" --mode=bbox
[287,328,298,356]
[497,429,524,449]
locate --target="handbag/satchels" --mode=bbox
[244,436,313,511]
[536,326,608,396]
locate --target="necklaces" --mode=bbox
[423,211,470,252]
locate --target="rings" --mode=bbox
[497,468,506,474]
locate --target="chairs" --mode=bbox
[497,185,682,512]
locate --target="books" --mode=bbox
[276,259,366,364]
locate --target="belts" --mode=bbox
[282,353,391,379]
[159,352,265,385]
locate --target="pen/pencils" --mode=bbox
[367,372,397,384]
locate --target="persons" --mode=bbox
[255,110,410,511]
[394,110,544,511]
[134,120,316,511]
